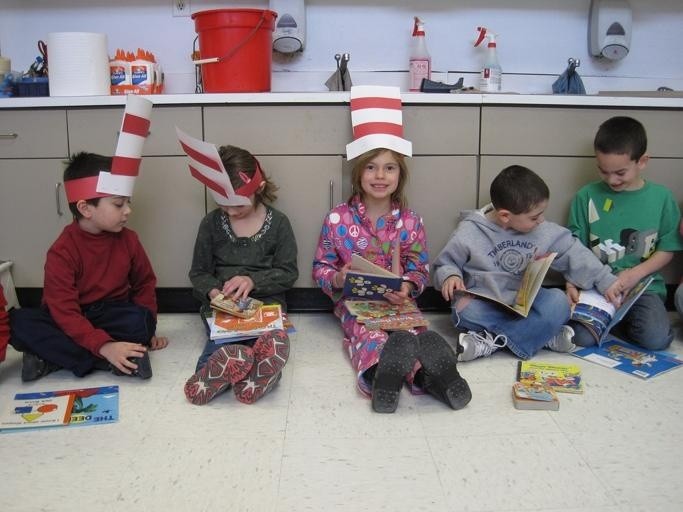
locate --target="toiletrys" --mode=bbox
[0,57,11,83]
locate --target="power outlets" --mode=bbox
[173,0,190,17]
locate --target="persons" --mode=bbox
[6,153,170,381]
[433,162,624,359]
[185,145,299,405]
[311,148,473,412]
[563,116,674,351]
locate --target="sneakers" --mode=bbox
[22,352,49,381]
[416,331,472,409]
[372,330,418,413]
[545,325,576,352]
[456,330,507,362]
[184,344,254,405]
[109,352,152,379]
[233,329,290,404]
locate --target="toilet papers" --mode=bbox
[45,33,112,96]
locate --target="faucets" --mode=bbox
[565,57,581,93]
[335,53,350,89]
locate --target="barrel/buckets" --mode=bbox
[191,7,279,93]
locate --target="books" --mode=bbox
[511,354,581,412]
[579,339,682,380]
[567,268,654,344]
[195,292,295,347]
[0,384,122,434]
[335,247,430,333]
[448,251,557,317]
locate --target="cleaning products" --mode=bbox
[471,26,503,93]
[109,48,163,93]
[407,14,431,90]
